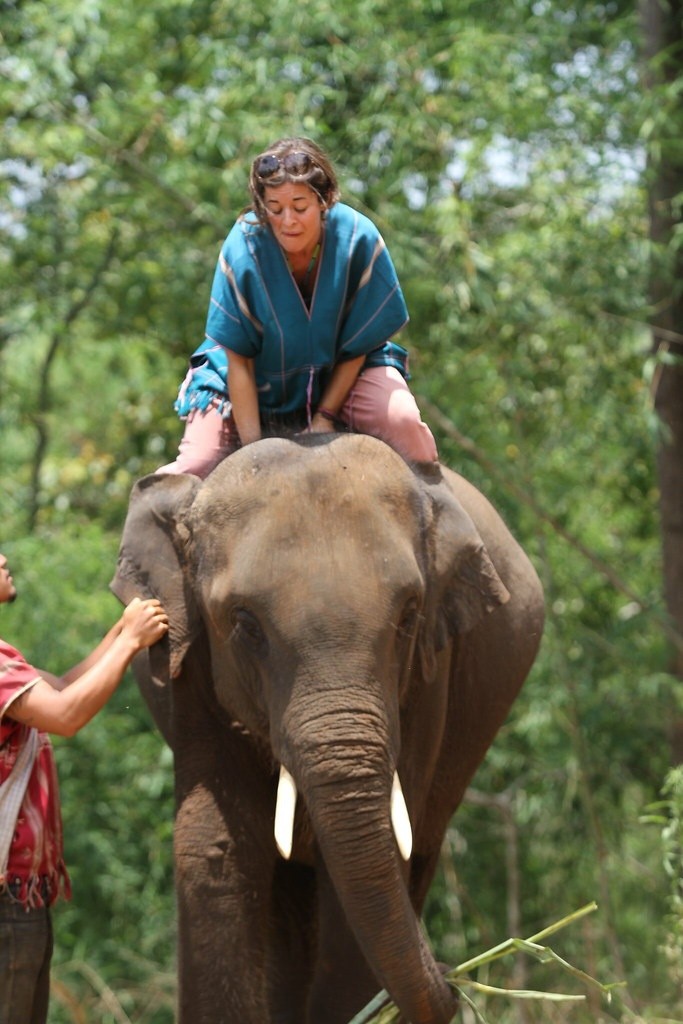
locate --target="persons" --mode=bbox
[152,138,438,481]
[0,552,169,1024]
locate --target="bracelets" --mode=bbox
[317,407,337,420]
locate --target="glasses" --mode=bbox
[254,153,320,179]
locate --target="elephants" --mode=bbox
[109,431,548,1024]
